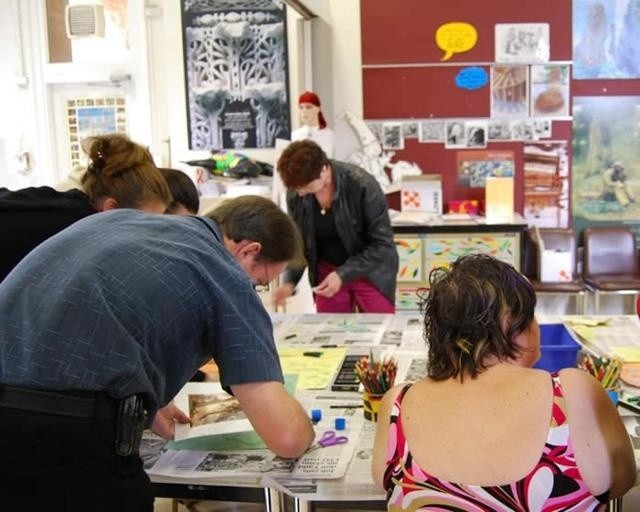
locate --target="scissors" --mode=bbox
[306,431,349,453]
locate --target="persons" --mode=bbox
[0,133,173,283]
[602,161,637,207]
[371,253,640,512]
[291,91,338,158]
[0,195,315,512]
[270,140,399,313]
[157,168,200,216]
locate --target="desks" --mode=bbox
[138,310,639,512]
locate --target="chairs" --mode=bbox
[522,225,640,315]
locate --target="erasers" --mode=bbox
[335,417,345,430]
[312,410,321,421]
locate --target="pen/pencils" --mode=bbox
[356,349,399,393]
[320,344,340,348]
[285,334,297,340]
[330,405,365,408]
[579,353,624,387]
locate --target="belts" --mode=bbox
[0,385,118,420]
